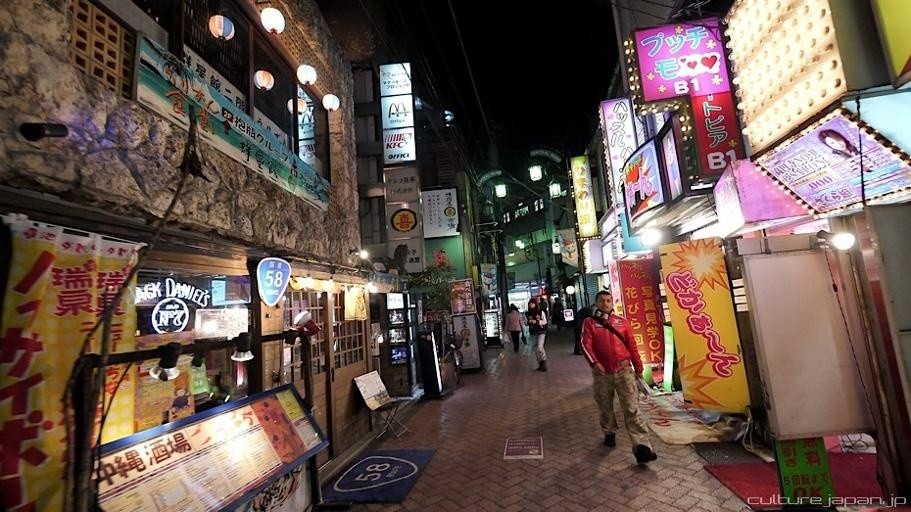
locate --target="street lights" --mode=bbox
[528,145,591,322]
[476,165,510,342]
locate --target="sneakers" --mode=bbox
[605,433,616,446]
[635,445,657,463]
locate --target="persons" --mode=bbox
[574,303,598,356]
[819,128,862,158]
[505,303,525,353]
[525,298,549,371]
[539,297,550,336]
[580,289,659,462]
[552,297,566,333]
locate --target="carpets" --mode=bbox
[316,448,438,503]
[703,450,888,511]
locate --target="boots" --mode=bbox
[537,360,547,371]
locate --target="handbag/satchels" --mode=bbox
[521,331,527,344]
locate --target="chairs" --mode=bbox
[350,370,413,441]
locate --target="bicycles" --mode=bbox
[443,331,465,386]
